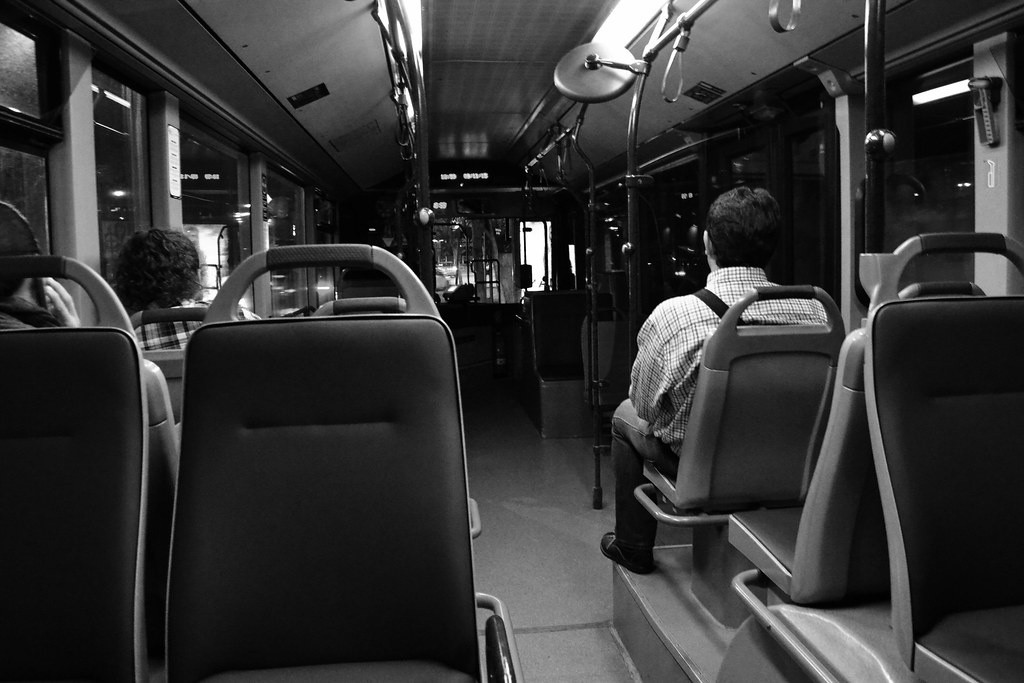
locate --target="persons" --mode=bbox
[111,227,262,350]
[1,202,83,328]
[601,188,828,575]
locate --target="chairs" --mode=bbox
[0,230,1024,683]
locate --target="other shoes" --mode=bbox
[598,532,655,574]
